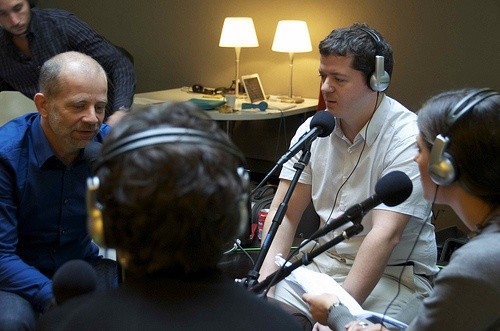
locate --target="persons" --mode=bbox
[257,21,440,331]
[0,0,136,128]
[0,52,122,331]
[304,87,500,331]
[28,101,305,331]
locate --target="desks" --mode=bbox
[132,86,319,146]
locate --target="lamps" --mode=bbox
[271,20,313,104]
[218,17,259,101]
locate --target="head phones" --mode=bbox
[84,129,251,248]
[429,88,496,185]
[359,26,390,92]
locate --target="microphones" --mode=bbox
[310,171,414,240]
[277,110,335,166]
[51,260,97,303]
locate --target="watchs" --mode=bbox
[329,302,343,314]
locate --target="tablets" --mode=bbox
[188,98,226,111]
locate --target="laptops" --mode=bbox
[241,74,296,111]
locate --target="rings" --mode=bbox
[362,323,369,328]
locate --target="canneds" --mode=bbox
[258,209,269,239]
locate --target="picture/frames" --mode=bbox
[240,74,267,103]
